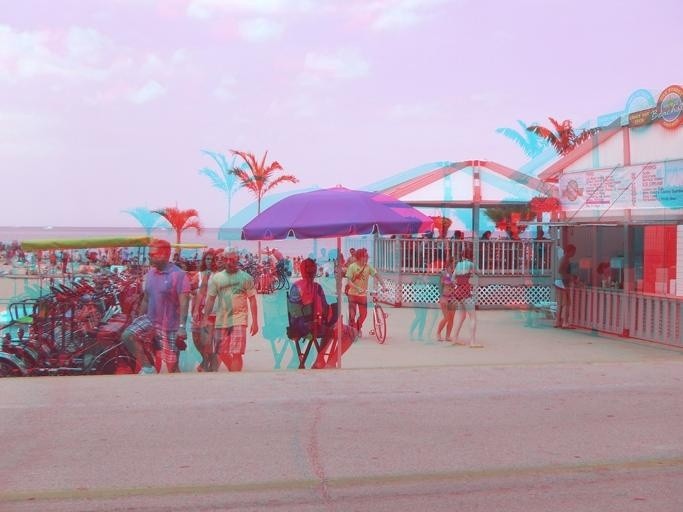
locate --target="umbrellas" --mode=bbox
[240,184,436,370]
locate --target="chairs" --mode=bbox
[286,291,333,369]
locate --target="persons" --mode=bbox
[596,261,617,288]
[553,243,581,329]
[0,228,552,373]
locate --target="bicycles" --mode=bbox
[241,259,289,293]
[362,288,388,343]
